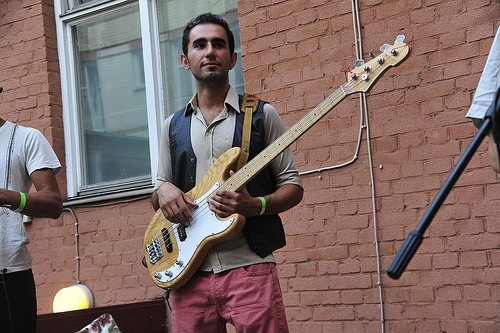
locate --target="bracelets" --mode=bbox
[13,191,26,213]
[258,197,266,216]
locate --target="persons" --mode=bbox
[0,87,63,333]
[151,12,303,333]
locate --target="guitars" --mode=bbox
[142,34,411,290]
